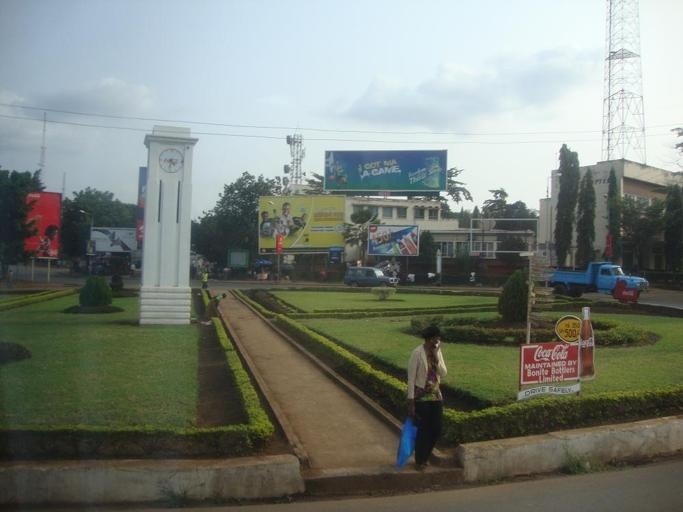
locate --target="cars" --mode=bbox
[91,257,135,277]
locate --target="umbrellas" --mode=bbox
[254,258,272,274]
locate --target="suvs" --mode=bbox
[344,266,398,288]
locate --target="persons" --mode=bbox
[405,326,448,470]
[213,294,227,309]
[259,202,309,241]
[201,271,209,289]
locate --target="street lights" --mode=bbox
[80,210,93,275]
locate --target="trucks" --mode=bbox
[543,261,649,301]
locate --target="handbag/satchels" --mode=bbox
[396,416,417,467]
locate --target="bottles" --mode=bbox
[577,307,598,383]
